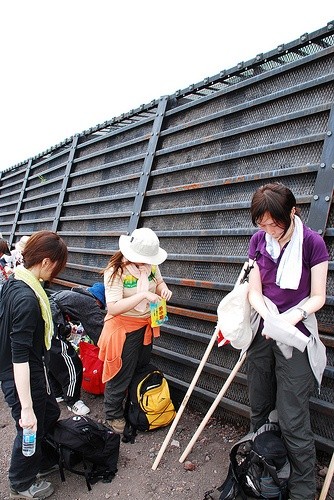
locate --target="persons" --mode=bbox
[0,231,71,500]
[236,182,329,500]
[0,238,16,292]
[99,228,172,433]
[3,234,32,275]
[41,281,112,418]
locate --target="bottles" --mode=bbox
[22,428,36,456]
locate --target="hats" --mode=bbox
[88,282,106,307]
[119,227,167,265]
[217,283,252,349]
[15,236,30,251]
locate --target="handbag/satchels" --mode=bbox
[77,341,106,395]
[60,323,72,338]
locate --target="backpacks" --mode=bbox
[124,364,178,432]
[228,423,290,500]
[55,414,120,491]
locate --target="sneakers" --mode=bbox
[37,464,60,476]
[55,396,63,403]
[10,478,54,500]
[67,400,90,416]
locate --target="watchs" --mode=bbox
[296,307,308,322]
